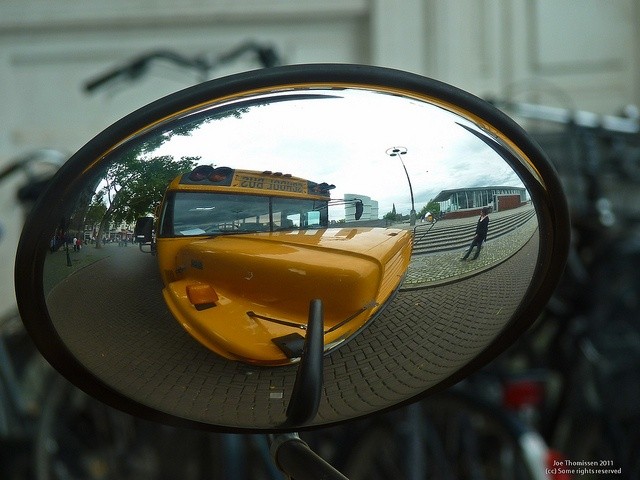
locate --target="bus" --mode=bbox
[135,166,437,370]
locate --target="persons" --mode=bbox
[76,239,81,251]
[461,208,490,261]
[73,236,77,251]
